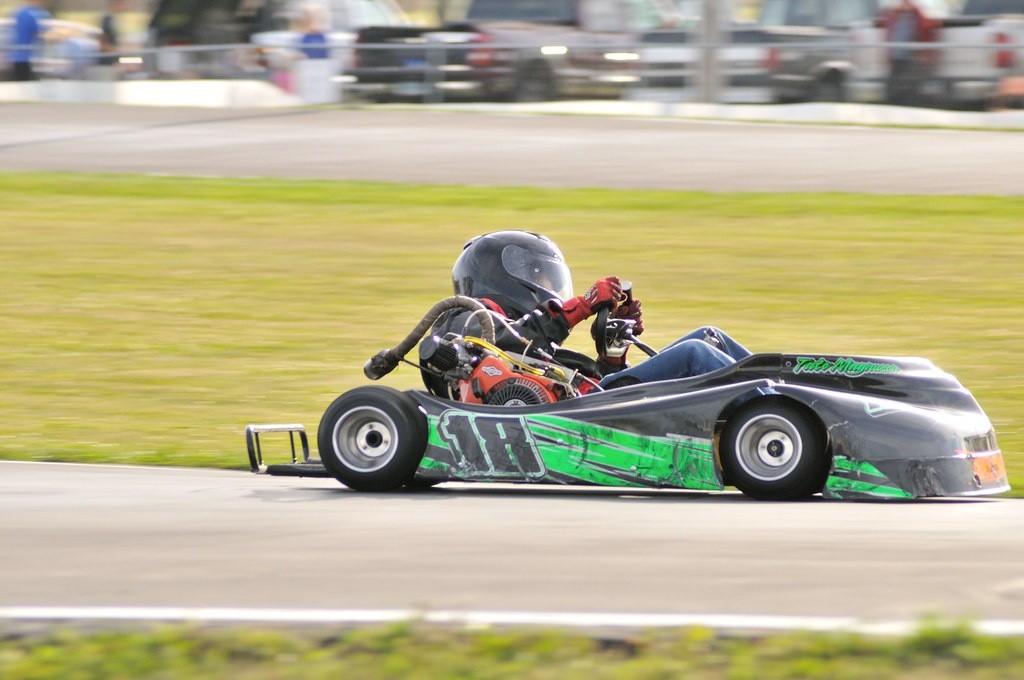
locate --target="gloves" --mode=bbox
[562,275,619,325]
[594,296,645,360]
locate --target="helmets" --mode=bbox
[452,229,574,315]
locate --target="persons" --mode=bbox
[418,229,645,408]
[0,0,1024,129]
